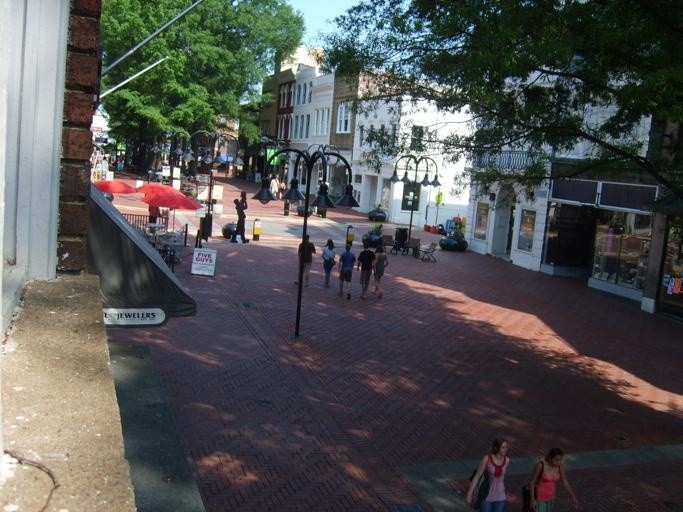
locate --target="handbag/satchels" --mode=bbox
[470,468,490,511]
[521,484,536,512]
[325,257,336,267]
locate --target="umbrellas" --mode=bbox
[136,184,186,197]
[139,189,206,234]
[93,181,139,194]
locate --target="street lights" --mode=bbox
[150,129,194,188]
[251,139,360,339]
[389,154,441,242]
[181,131,246,237]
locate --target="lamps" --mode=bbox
[283,161,288,177]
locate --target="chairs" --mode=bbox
[382,234,397,255]
[401,239,421,258]
[420,241,437,264]
[444,219,454,235]
[131,218,188,272]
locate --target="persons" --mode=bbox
[466,437,511,511]
[356,243,375,300]
[266,173,285,201]
[149,203,160,233]
[230,198,248,243]
[338,245,355,298]
[372,247,388,298]
[527,446,580,512]
[321,238,336,288]
[294,234,316,287]
